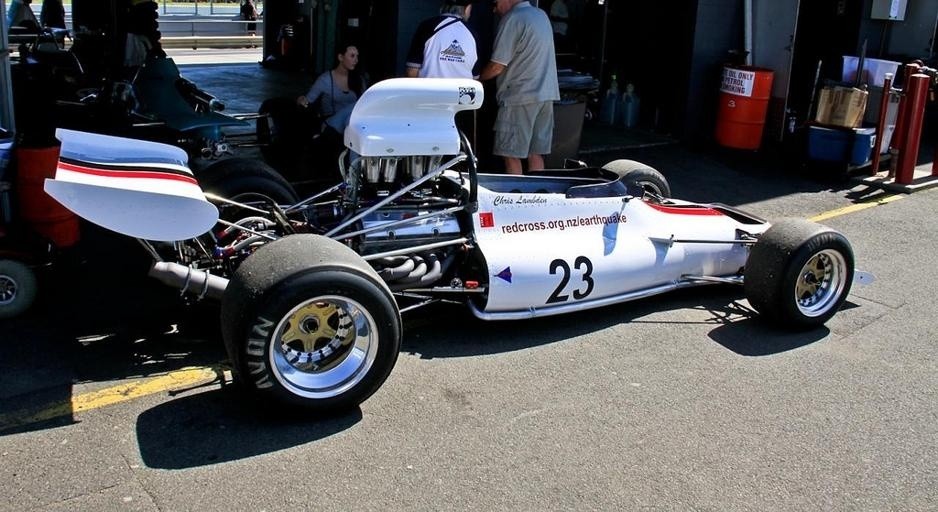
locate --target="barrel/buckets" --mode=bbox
[714,64,774,150]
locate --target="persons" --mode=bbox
[241,1,257,36]
[276,8,309,85]
[478,1,561,174]
[406,0,488,80]
[295,40,367,180]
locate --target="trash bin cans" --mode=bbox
[545,70,600,169]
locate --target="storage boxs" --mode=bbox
[805,55,903,167]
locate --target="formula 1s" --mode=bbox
[41,76,856,426]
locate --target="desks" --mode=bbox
[163,112,250,156]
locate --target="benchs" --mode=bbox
[255,81,367,181]
